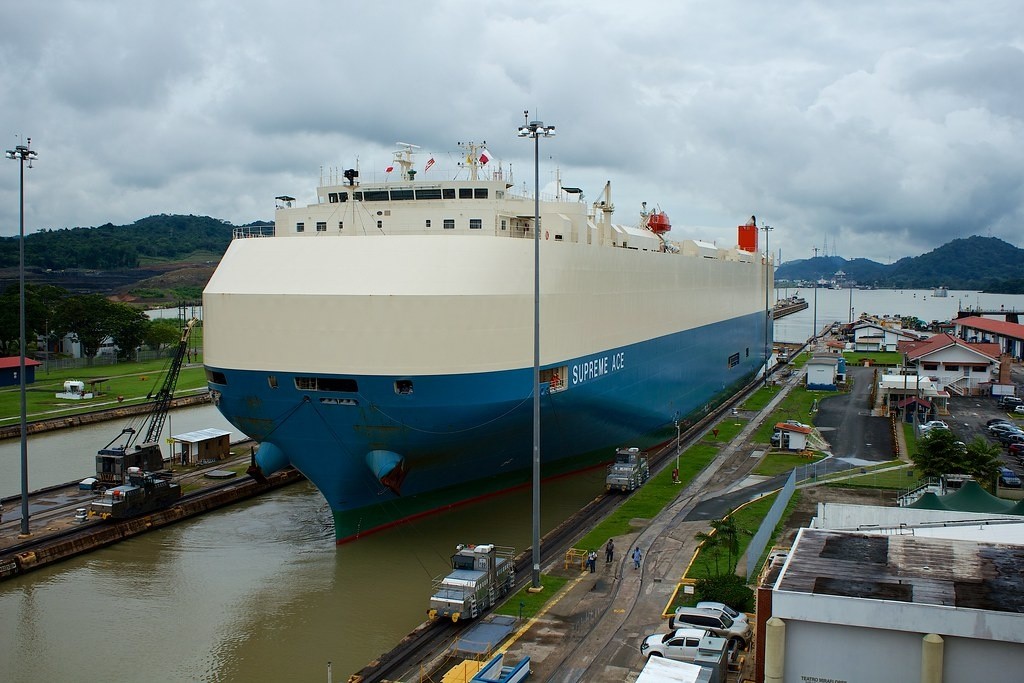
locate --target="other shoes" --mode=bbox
[591,571,595,573]
[606,561,612,563]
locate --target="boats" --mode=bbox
[203,143,775,552]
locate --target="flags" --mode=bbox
[479,149,494,165]
[425,158,435,172]
[466,153,472,164]
[385,167,393,172]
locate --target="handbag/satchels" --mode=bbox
[586,560,590,565]
[632,554,634,559]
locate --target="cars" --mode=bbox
[768,550,788,566]
[987,418,1024,454]
[997,468,1021,488]
[770,433,789,447]
[519,113,557,591]
[917,420,949,438]
[999,396,1024,414]
[697,602,749,624]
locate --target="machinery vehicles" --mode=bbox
[427,544,516,623]
[606,447,649,492]
[88,467,181,518]
[96,318,198,485]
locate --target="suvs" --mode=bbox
[668,606,752,652]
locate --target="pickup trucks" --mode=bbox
[640,628,738,664]
[773,419,812,433]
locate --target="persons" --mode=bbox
[549,373,561,388]
[605,538,614,563]
[588,548,598,573]
[182,450,189,467]
[397,384,413,394]
[631,546,642,570]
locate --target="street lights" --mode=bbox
[5,133,36,541]
[761,222,777,388]
[673,421,680,483]
[813,249,820,343]
